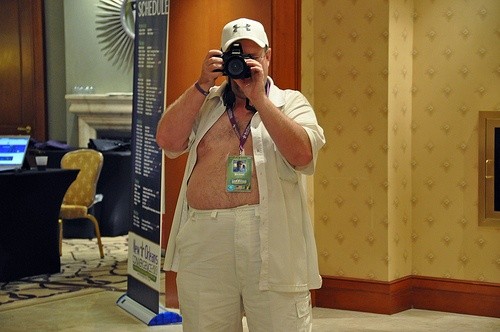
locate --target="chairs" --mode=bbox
[59,149,104,258]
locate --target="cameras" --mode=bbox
[212,42,252,79]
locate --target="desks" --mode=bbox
[0,168,81,283]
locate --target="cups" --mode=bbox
[35,156,48,171]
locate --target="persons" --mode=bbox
[155,18,326,332]
[233,161,245,172]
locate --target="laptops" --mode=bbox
[0,134,31,172]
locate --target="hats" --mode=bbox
[221,17,269,51]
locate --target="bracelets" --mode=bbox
[195,82,210,96]
